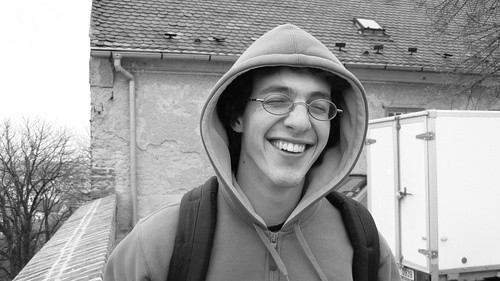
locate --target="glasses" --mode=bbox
[247,93,343,121]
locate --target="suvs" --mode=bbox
[366,108,500,281]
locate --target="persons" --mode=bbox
[102,23,405,281]
